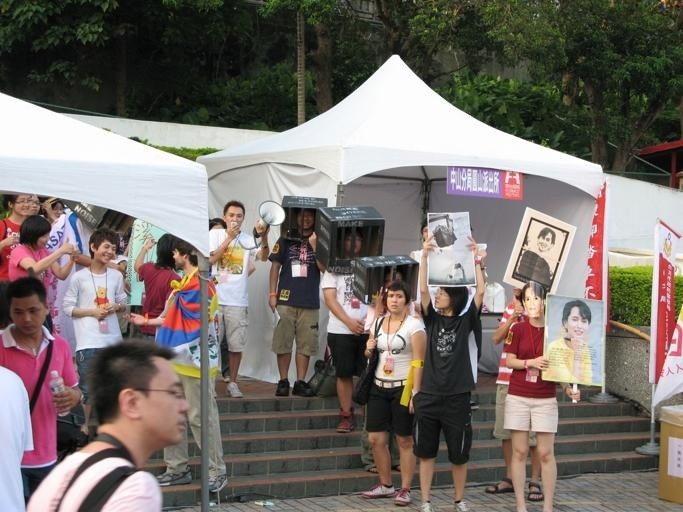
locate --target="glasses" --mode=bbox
[134,385,185,399]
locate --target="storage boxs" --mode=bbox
[659,404,682,505]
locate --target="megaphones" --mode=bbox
[252,199,288,239]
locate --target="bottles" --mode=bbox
[47,370,71,418]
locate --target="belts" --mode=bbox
[374,378,407,388]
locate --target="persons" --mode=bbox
[0,185,256,503]
[25,338,191,512]
[0,362,35,512]
[542,301,596,386]
[509,213,556,292]
[207,194,559,512]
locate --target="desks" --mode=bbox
[476,312,505,376]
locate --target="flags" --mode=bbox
[582,182,611,335]
[650,303,683,408]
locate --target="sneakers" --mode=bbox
[156,465,192,485]
[275,379,289,396]
[208,474,228,492]
[292,380,313,397]
[336,400,544,512]
[226,382,243,397]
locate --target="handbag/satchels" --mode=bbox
[307,363,336,398]
[352,349,378,406]
